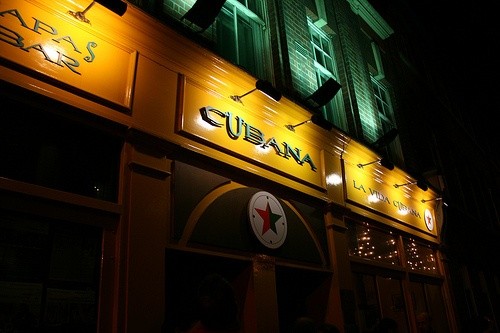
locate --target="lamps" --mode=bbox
[421,194,449,207]
[285,114,332,132]
[357,157,394,171]
[393,179,428,191]
[231,79,285,104]
[67,0,128,25]
